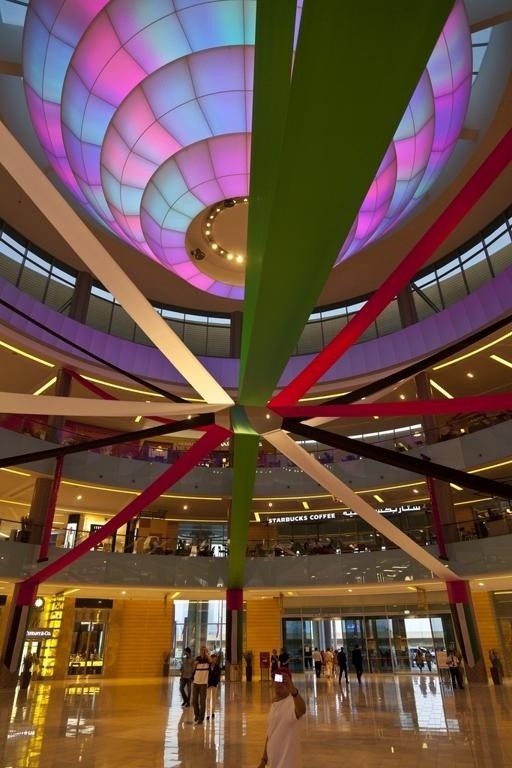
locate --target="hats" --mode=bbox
[271,666,292,680]
[184,647,191,653]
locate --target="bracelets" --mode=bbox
[261,758,267,764]
[291,688,298,697]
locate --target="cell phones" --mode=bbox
[274,674,283,684]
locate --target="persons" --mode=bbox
[352,644,363,685]
[271,648,278,686]
[304,644,313,670]
[177,538,354,557]
[278,648,290,668]
[179,647,221,725]
[314,648,339,679]
[337,647,349,684]
[393,441,408,451]
[323,451,332,464]
[407,648,464,693]
[256,666,306,768]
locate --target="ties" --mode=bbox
[452,656,454,665]
